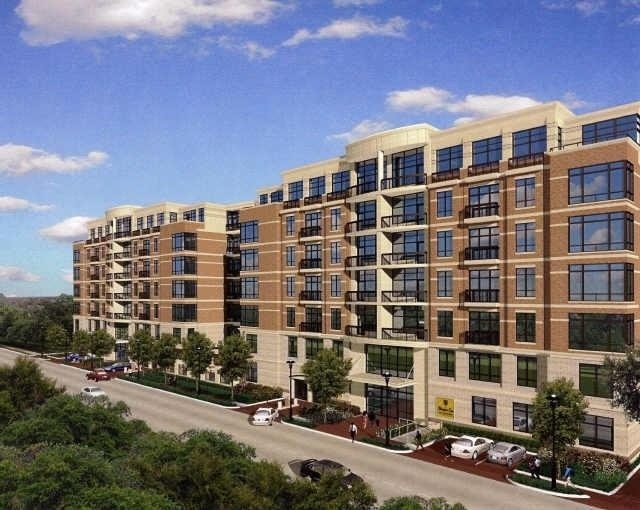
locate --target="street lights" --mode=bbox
[380,370,393,447]
[285,355,297,421]
[543,393,561,492]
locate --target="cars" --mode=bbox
[84,367,111,382]
[299,458,365,492]
[79,386,107,399]
[449,435,495,461]
[103,361,131,373]
[251,407,280,426]
[485,441,527,466]
